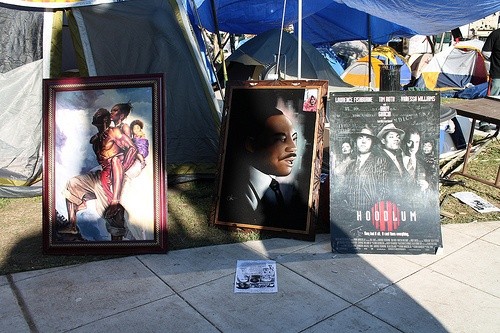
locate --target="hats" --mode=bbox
[351,127,379,143]
[377,123,406,140]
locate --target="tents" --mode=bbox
[0,0,228,199]
[216,25,500,101]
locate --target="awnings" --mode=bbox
[179,0,500,92]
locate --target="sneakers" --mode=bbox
[57,222,78,234]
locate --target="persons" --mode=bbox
[481,27,500,96]
[401,126,427,202]
[345,129,392,213]
[417,137,439,193]
[376,123,409,183]
[333,141,356,189]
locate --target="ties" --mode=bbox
[271,179,286,213]
[407,158,415,176]
[353,155,362,173]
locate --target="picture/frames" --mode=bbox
[207,79,327,242]
[42,72,168,256]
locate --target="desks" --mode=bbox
[439,94,500,192]
